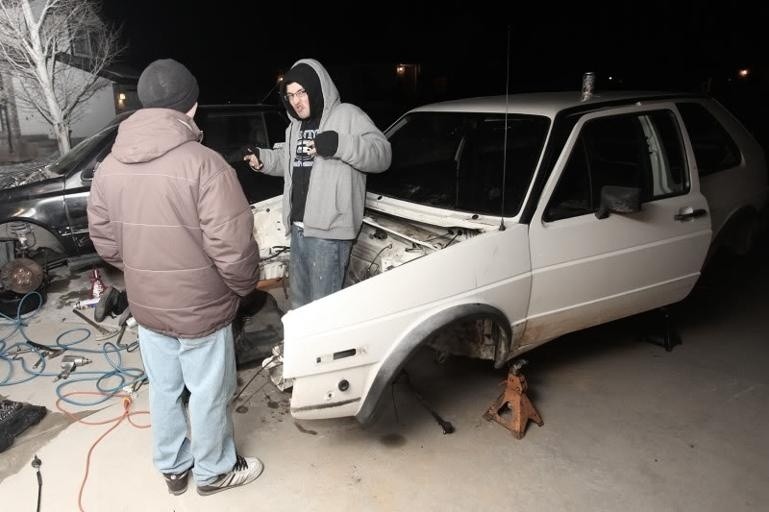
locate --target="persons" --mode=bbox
[241,57,397,312]
[86,55,265,497]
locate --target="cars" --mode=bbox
[0,103,288,288]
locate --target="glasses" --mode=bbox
[283,90,308,102]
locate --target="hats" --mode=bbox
[135,57,201,115]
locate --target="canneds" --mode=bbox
[581,71,595,99]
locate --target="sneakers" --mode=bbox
[196,455,265,498]
[161,468,191,497]
